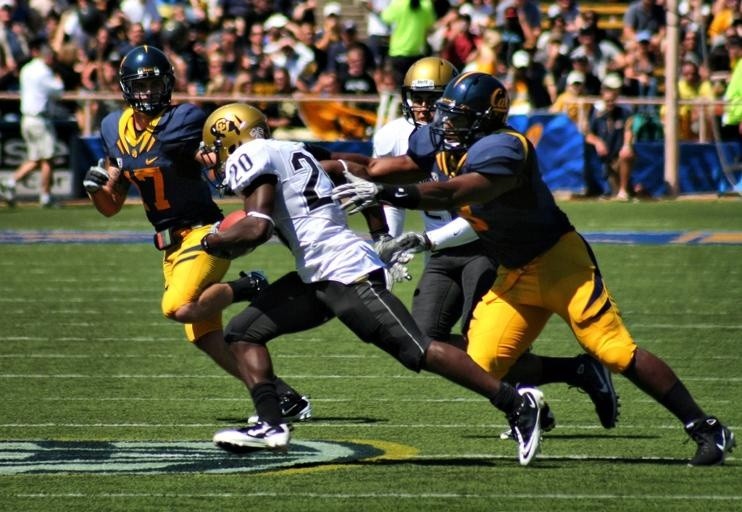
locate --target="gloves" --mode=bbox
[83,158,109,194]
[331,170,379,215]
[374,231,427,283]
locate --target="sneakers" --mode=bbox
[213,423,290,453]
[515,383,545,466]
[569,353,620,429]
[247,396,312,424]
[239,271,268,302]
[683,416,736,468]
[500,404,557,440]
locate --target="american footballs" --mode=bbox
[219,210,256,255]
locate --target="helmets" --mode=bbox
[429,72,510,152]
[119,45,175,112]
[401,56,459,128]
[199,103,271,190]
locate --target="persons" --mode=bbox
[0,0,742,214]
[370,56,622,430]
[198,102,546,468]
[82,46,311,428]
[330,74,738,467]
